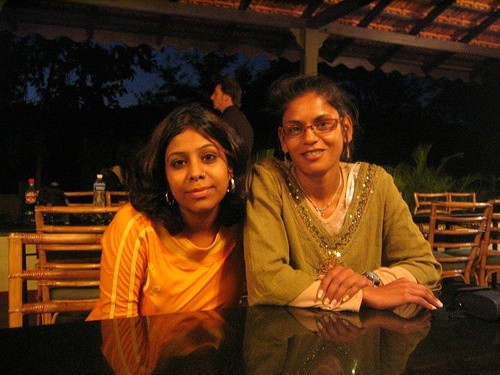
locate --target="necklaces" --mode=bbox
[291,167,342,216]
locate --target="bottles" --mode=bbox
[25,178,38,229]
[93,174,105,218]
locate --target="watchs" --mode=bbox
[361,271,379,285]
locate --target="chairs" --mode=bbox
[414,192,500,286]
[8,190,143,327]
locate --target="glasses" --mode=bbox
[282,117,344,139]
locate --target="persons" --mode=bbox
[85,105,253,321]
[210,79,251,158]
[244,76,443,310]
[99,302,433,374]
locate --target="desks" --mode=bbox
[13,202,117,229]
[0,287,500,375]
[413,211,500,224]
